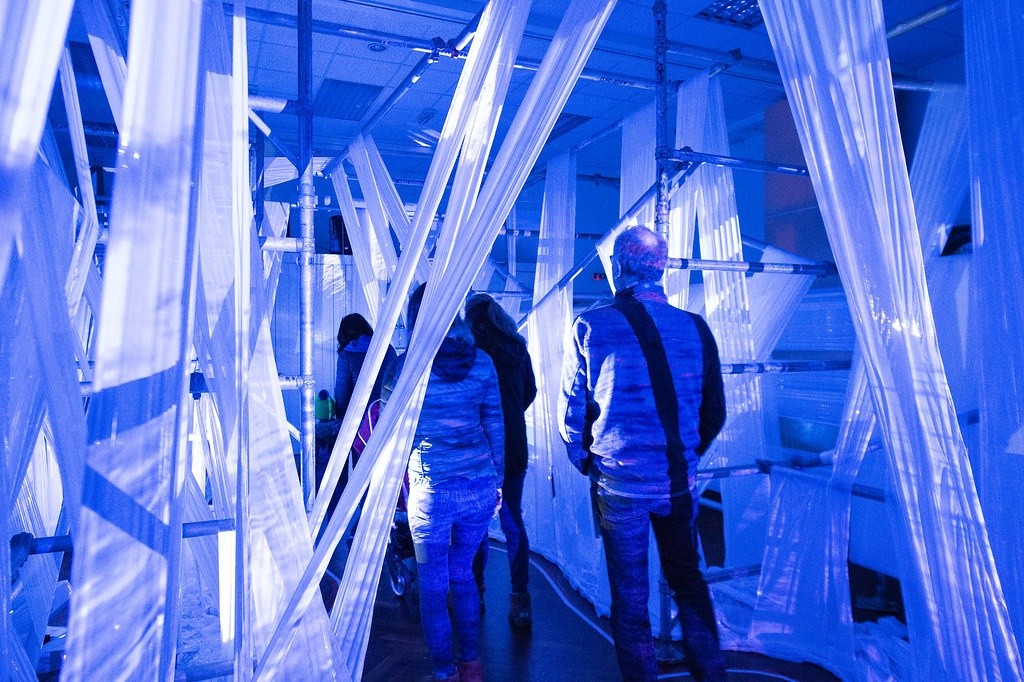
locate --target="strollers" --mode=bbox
[320,398,417,601]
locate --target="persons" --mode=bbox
[465,293,538,630]
[378,282,505,682]
[557,225,727,682]
[316,312,398,553]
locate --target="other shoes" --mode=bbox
[458,659,482,682]
[435,667,460,682]
[509,592,532,627]
[479,596,486,618]
